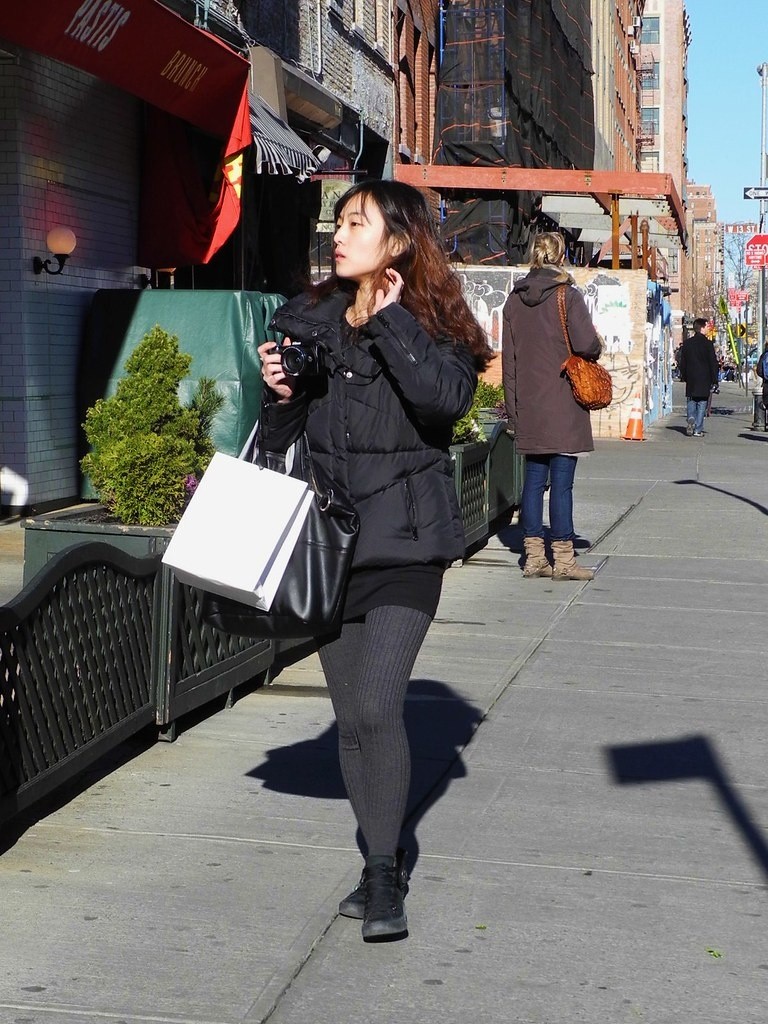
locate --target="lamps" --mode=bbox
[33,226,76,275]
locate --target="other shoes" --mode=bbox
[686,417,695,435]
[694,432,704,437]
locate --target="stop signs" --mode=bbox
[746,233,768,270]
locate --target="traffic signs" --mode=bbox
[743,187,768,201]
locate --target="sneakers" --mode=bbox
[338,848,407,917]
[361,854,407,939]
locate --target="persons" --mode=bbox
[756,341,768,411]
[680,318,719,436]
[502,231,602,581]
[256,181,498,940]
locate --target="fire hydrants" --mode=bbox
[751,380,768,432]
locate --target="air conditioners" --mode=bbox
[630,40,636,50]
[628,26,634,37]
[632,16,640,28]
[632,45,639,55]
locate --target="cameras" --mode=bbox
[268,341,324,377]
[710,385,720,394]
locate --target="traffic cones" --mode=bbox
[621,392,647,441]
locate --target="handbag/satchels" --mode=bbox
[557,285,613,410]
[201,426,360,639]
[161,418,315,613]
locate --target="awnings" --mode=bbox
[248,91,321,184]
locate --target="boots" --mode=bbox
[551,540,594,581]
[521,538,554,579]
